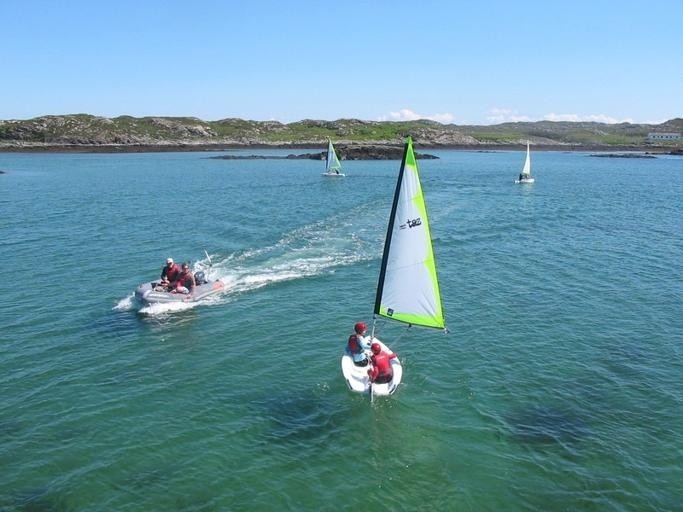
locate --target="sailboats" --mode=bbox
[321,138,346,177]
[514,141,535,184]
[341,136,446,396]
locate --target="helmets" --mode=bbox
[371,343,381,351]
[355,322,368,331]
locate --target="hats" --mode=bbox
[166,258,173,264]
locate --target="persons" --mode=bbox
[344,322,371,367]
[368,343,398,383]
[160,258,196,299]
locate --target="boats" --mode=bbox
[135,269,225,306]
